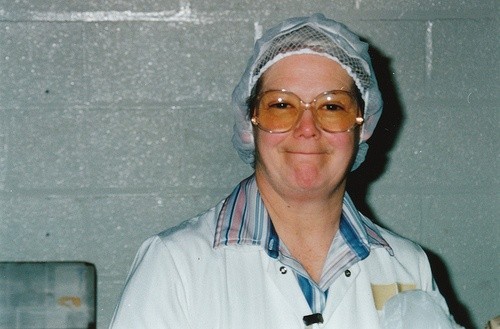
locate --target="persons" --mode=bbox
[109,13,464,328]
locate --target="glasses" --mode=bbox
[251,90,364,134]
